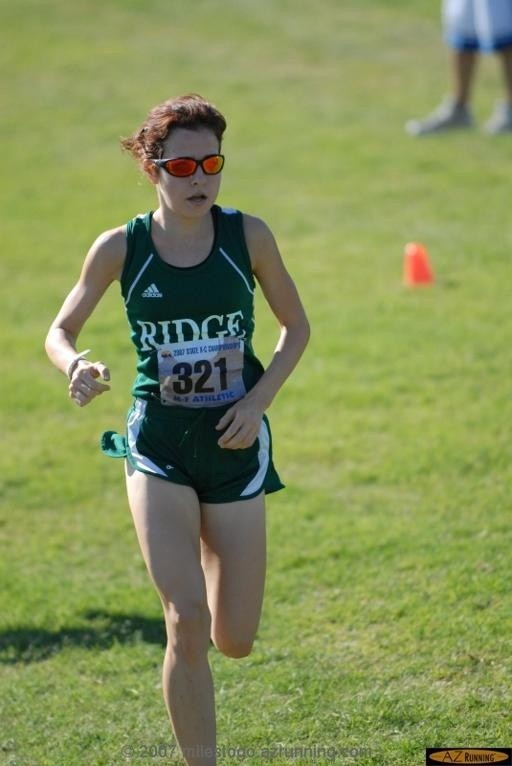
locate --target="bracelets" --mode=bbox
[68,349,90,380]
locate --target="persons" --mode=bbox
[406,1,512,135]
[44,94,309,766]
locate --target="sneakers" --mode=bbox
[405,100,512,137]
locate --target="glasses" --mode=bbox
[149,154,225,177]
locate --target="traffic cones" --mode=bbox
[401,238,434,289]
